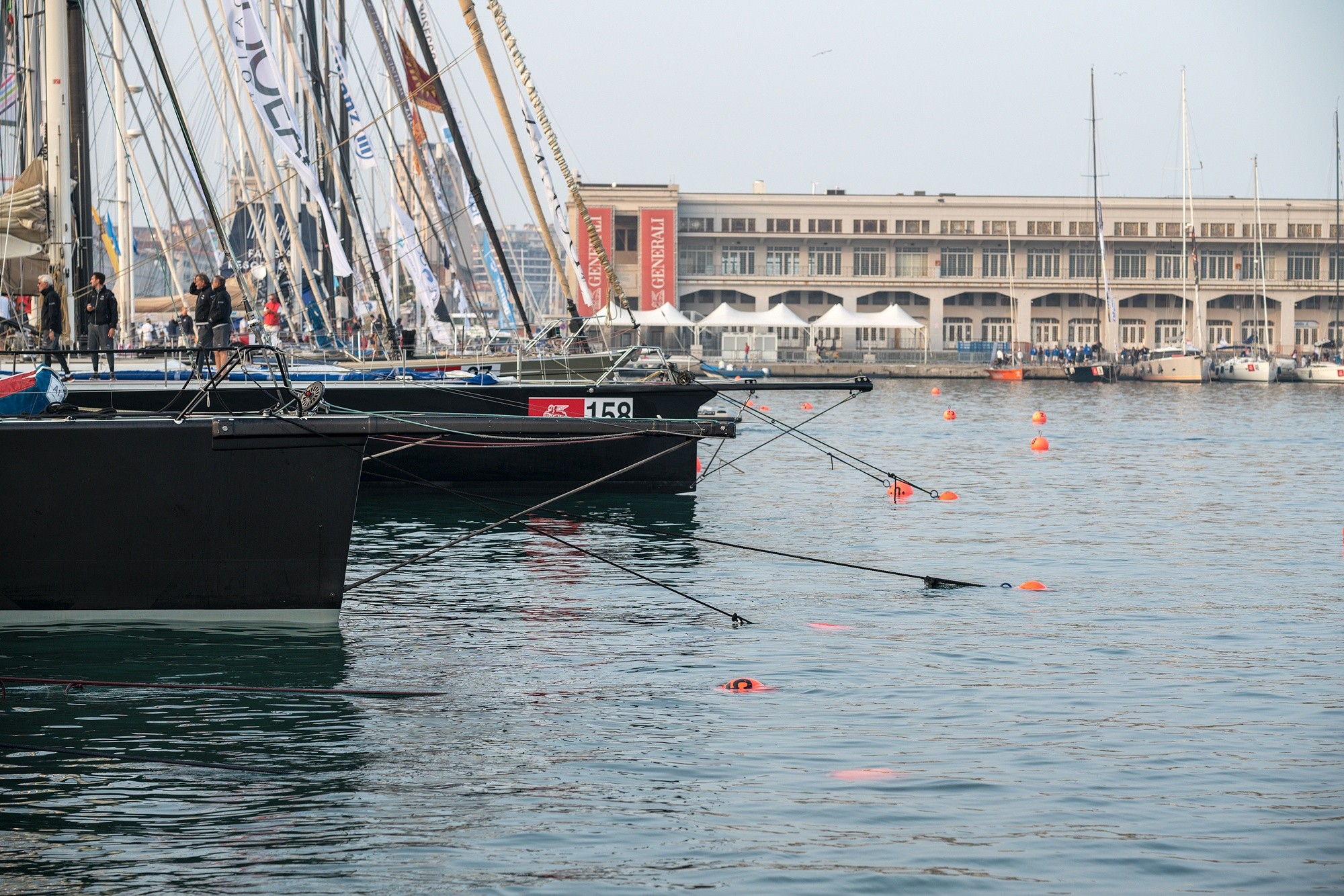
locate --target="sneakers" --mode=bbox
[60,375,75,382]
[89,373,101,380]
[109,373,117,380]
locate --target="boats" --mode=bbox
[1,346,379,634]
[698,364,772,379]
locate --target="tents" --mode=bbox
[582,301,927,365]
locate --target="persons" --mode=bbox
[263,293,281,358]
[1291,349,1330,368]
[140,307,195,349]
[38,274,75,383]
[347,314,403,358]
[996,348,1023,368]
[84,272,118,383]
[5,326,15,357]
[744,342,749,363]
[1030,342,1103,366]
[1241,348,1246,357]
[1121,345,1150,367]
[816,344,821,362]
[240,315,310,343]
[190,273,232,382]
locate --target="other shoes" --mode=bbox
[190,374,204,381]
[208,375,229,382]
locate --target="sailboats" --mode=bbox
[1292,110,1344,385]
[1132,67,1214,383]
[1058,69,1123,384]
[984,222,1032,381]
[1212,154,1283,382]
[0,1,876,493]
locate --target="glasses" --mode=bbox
[38,283,44,285]
[269,298,275,300]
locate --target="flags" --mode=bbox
[396,32,444,176]
[105,211,121,255]
[133,234,140,255]
